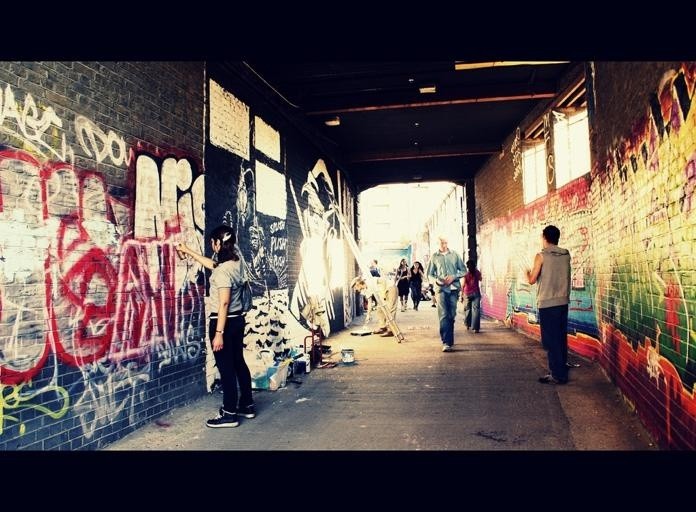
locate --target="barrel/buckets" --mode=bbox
[341,349,355,363]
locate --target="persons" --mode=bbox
[177,221,256,427]
[526,224,570,385]
[428,234,467,353]
[349,254,485,337]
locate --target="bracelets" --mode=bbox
[215,329,225,335]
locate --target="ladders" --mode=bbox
[323,184,403,342]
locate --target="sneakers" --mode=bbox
[442,342,452,352]
[206,410,240,428]
[236,403,256,419]
[400,304,437,312]
[539,375,567,384]
